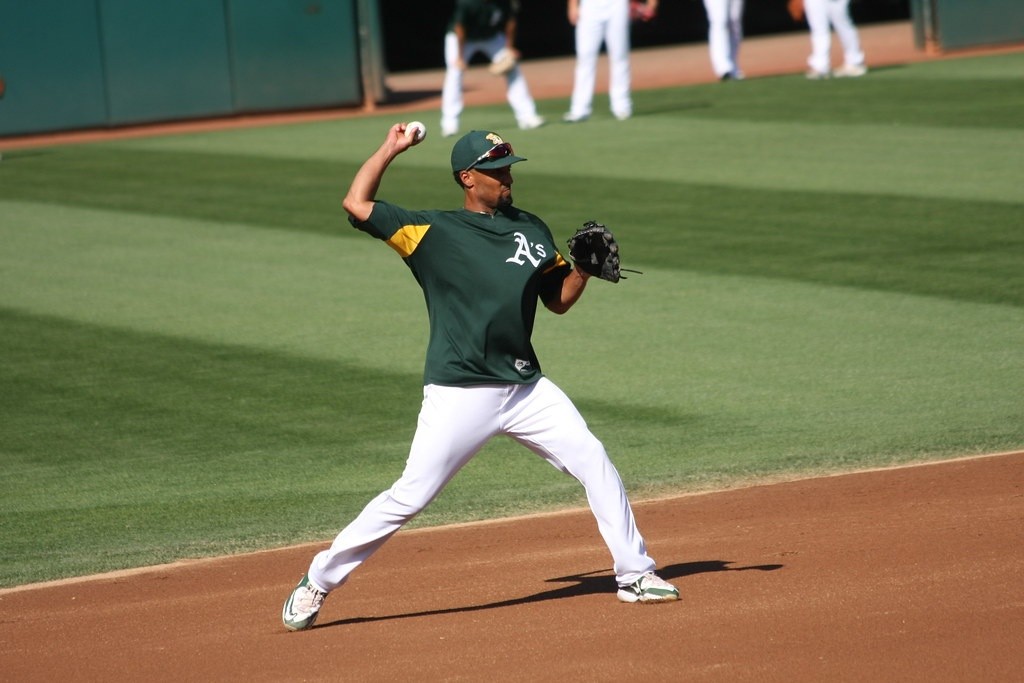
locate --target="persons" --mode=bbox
[803,1,867,80]
[282,122,680,631]
[563,1,658,121]
[441,0,546,137]
[704,0,743,81]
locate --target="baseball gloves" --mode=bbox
[787,0,805,22]
[565,219,646,285]
[489,50,516,75]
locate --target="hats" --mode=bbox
[451,130,527,171]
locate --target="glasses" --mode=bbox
[466,143,514,171]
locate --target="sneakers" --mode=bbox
[617,573,679,602]
[282,572,328,631]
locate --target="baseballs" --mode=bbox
[405,120,426,141]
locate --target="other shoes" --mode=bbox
[834,63,866,78]
[441,127,459,137]
[519,117,545,129]
[807,70,830,80]
[564,111,591,122]
